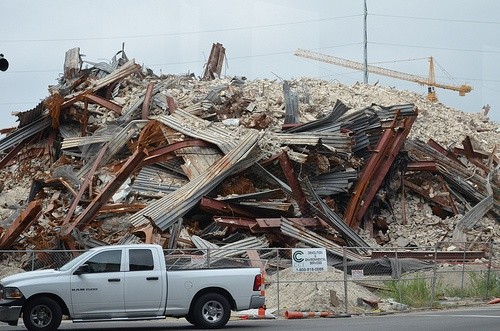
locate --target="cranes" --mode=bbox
[290,45,471,114]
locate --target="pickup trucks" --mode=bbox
[0,242,265,330]
